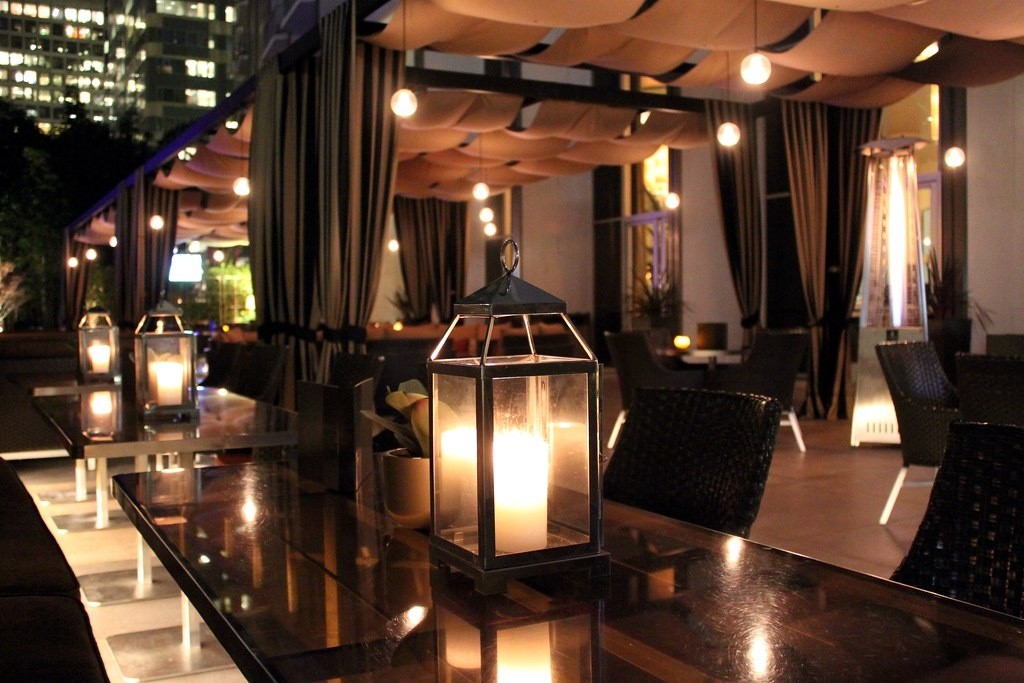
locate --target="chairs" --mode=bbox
[875,334,1024,619]
[203,340,289,403]
[601,388,783,539]
[605,323,811,452]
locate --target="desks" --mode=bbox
[112,455,1024,683]
[681,353,741,364]
[5,369,301,528]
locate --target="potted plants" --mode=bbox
[359,378,459,531]
[925,247,994,385]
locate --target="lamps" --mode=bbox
[717,52,741,147]
[472,133,490,201]
[426,238,612,601]
[390,0,418,117]
[144,424,197,525]
[740,0,772,85]
[78,384,123,442]
[433,573,605,683]
[233,125,251,195]
[77,300,122,382]
[132,291,200,424]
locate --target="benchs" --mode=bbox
[1,331,79,460]
[0,456,112,683]
